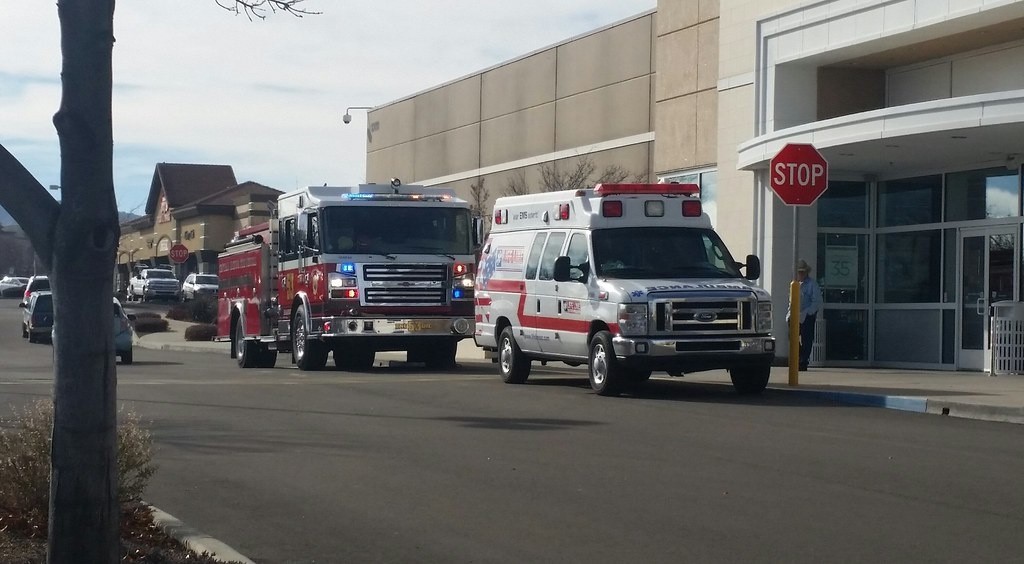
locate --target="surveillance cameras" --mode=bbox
[343,115,351,124]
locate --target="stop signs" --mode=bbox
[769,142,829,207]
[170,244,191,263]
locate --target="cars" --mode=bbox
[23,275,50,303]
[0,276,30,294]
[51,297,135,365]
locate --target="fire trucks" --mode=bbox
[214,182,482,368]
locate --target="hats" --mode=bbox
[798,260,812,273]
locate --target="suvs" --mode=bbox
[179,272,218,302]
[18,290,52,342]
[126,268,180,303]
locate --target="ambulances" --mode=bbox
[474,181,778,399]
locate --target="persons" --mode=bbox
[785,260,822,372]
[327,219,363,251]
[658,233,700,271]
